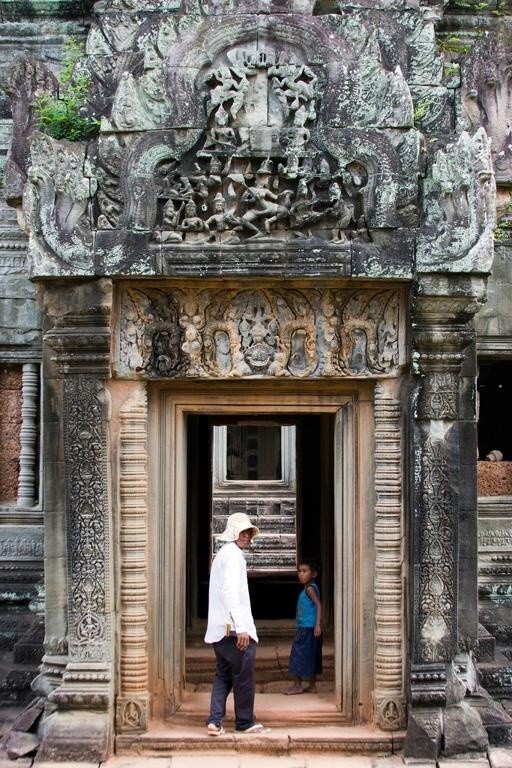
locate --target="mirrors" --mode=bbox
[217,426,289,488]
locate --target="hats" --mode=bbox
[214,514,259,542]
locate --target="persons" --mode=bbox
[283,555,323,695]
[204,512,271,737]
[154,59,369,245]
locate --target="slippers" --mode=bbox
[232,722,271,733]
[208,724,226,736]
